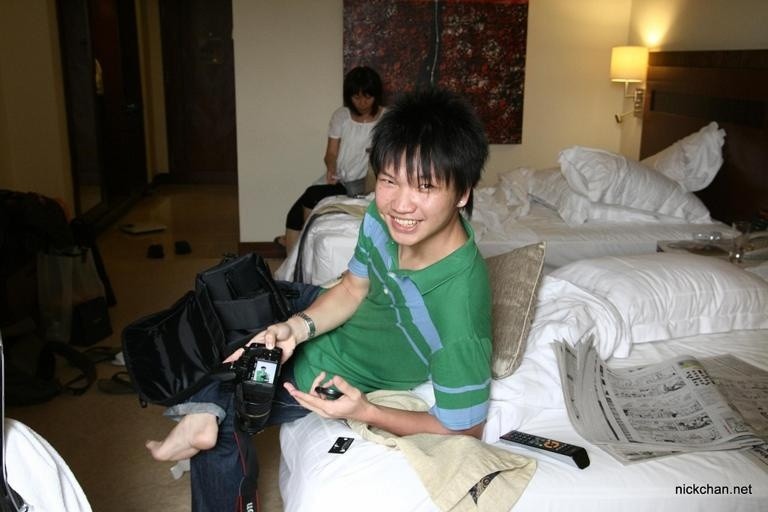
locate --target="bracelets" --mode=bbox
[291,309,317,343]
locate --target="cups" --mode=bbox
[732,221,751,249]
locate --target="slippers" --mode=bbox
[98,370,134,394]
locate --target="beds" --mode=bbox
[273,49,768,287]
[276,269,768,512]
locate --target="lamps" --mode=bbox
[610,47,648,123]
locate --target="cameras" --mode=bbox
[210,341,283,436]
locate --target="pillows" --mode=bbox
[744,259,768,283]
[549,253,768,345]
[497,166,660,229]
[484,240,546,378]
[557,145,714,225]
[636,121,727,193]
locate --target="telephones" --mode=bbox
[734,230,768,259]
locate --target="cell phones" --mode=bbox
[315,386,343,399]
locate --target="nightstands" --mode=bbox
[657,240,768,269]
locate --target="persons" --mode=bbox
[255,366,271,383]
[273,67,392,258]
[142,81,495,511]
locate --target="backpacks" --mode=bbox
[120,249,291,410]
[2,314,96,406]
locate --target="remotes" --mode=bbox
[499,429,590,470]
